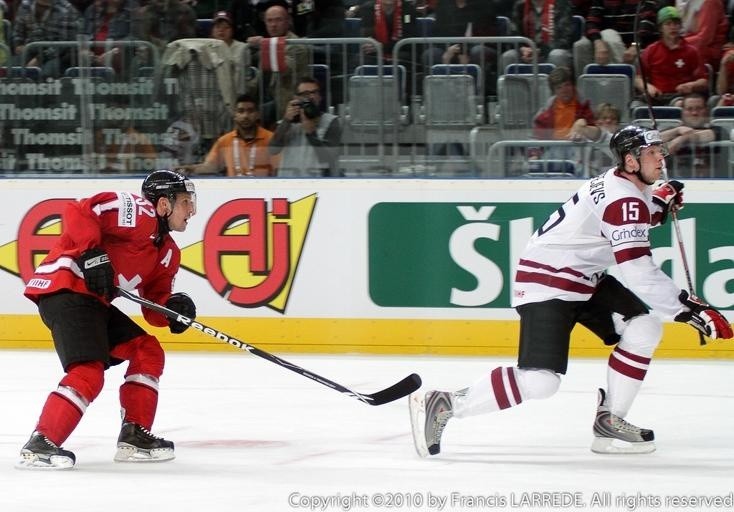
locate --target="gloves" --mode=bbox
[166,292,196,334]
[652,180,684,225]
[674,289,733,341]
[75,247,115,297]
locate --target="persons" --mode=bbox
[1,1,732,178]
[20,170,196,465]
[424,124,734,455]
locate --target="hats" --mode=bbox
[656,6,682,26]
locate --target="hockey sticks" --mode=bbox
[109,286,422,406]
[634,0,705,346]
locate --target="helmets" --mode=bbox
[141,168,197,219]
[610,125,670,173]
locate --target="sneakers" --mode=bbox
[14,430,75,468]
[116,407,174,452]
[424,387,470,455]
[593,388,654,442]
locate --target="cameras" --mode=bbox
[289,99,322,124]
